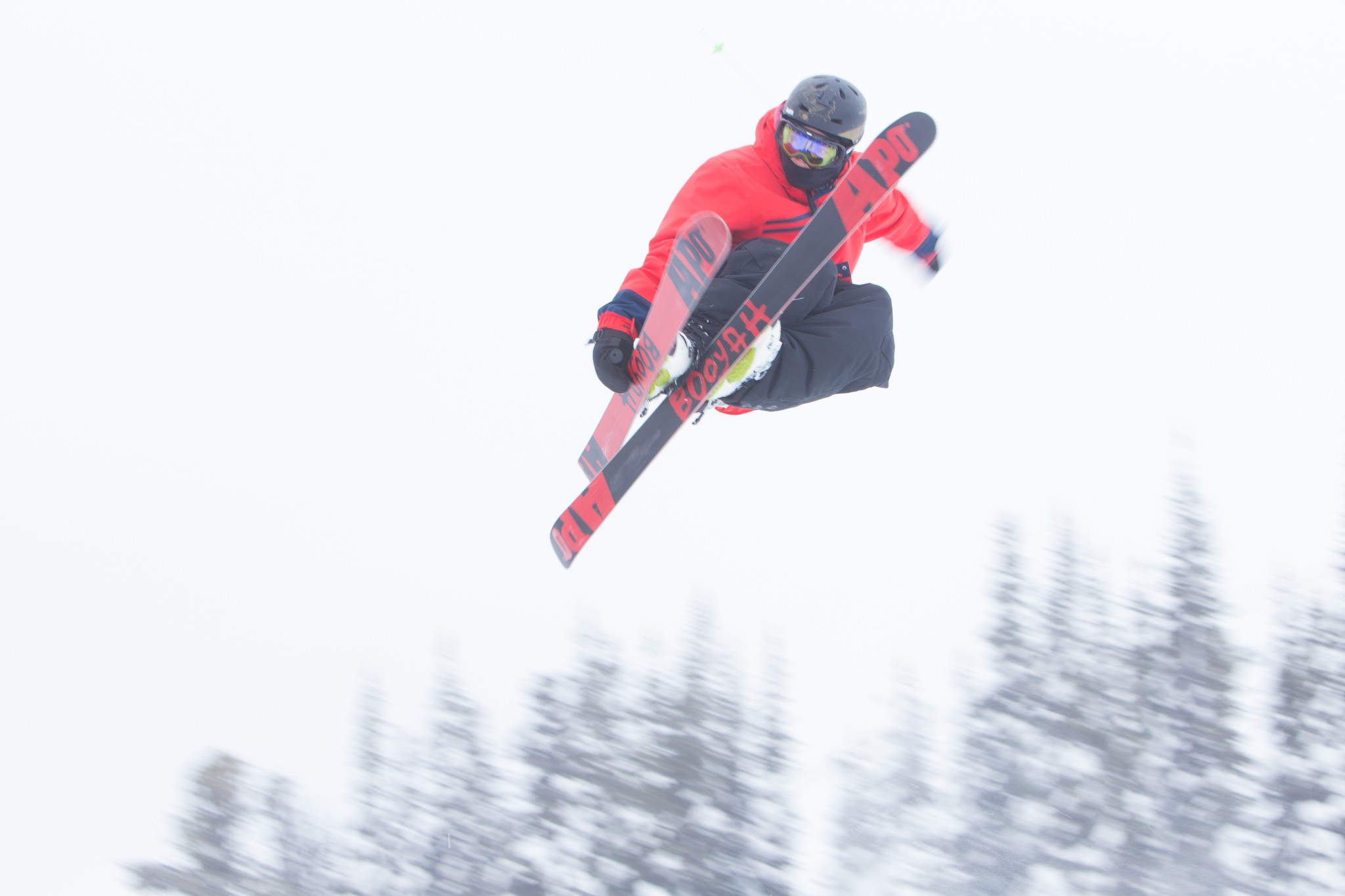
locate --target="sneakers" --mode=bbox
[628,324,695,405]
[703,319,785,402]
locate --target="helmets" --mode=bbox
[780,72,868,149]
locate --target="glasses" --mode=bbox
[773,116,850,170]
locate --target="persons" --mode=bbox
[583,73,942,424]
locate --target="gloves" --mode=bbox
[589,333,631,394]
[915,230,942,274]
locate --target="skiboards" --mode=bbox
[548,109,938,569]
